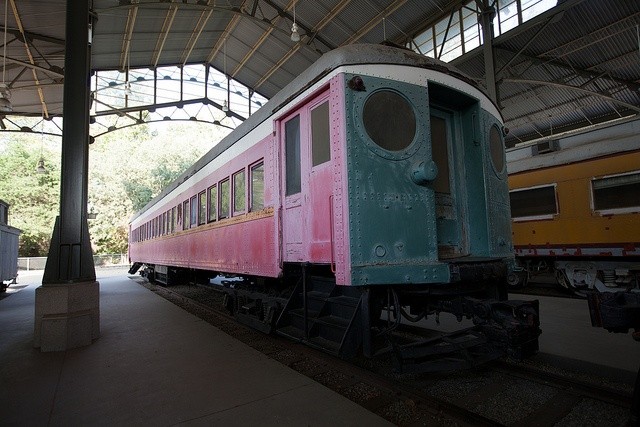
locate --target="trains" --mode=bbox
[129,43,539,358]
[505,114,640,299]
